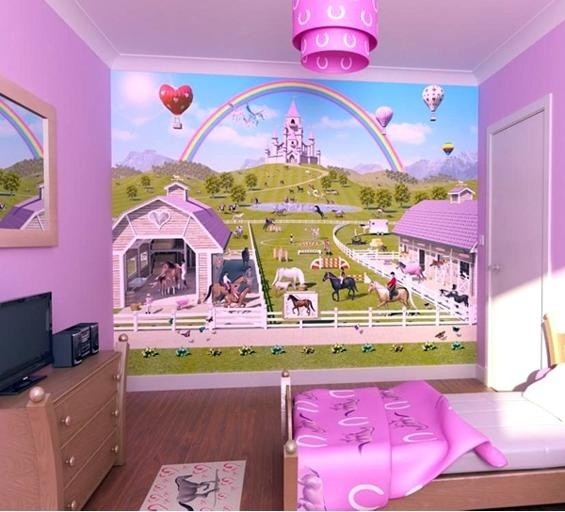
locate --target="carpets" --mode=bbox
[135,457,252,511]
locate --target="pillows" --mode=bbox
[519,359,565,424]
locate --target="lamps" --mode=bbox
[288,1,385,76]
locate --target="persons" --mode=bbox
[338,267,347,284]
[386,272,397,299]
[223,273,231,292]
[145,293,152,313]
[176,260,190,290]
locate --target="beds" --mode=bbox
[276,308,565,510]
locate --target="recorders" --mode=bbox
[51,322,99,367]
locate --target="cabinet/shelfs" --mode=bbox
[0,331,131,510]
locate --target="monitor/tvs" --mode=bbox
[1,291,55,396]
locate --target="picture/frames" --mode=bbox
[0,71,59,248]
